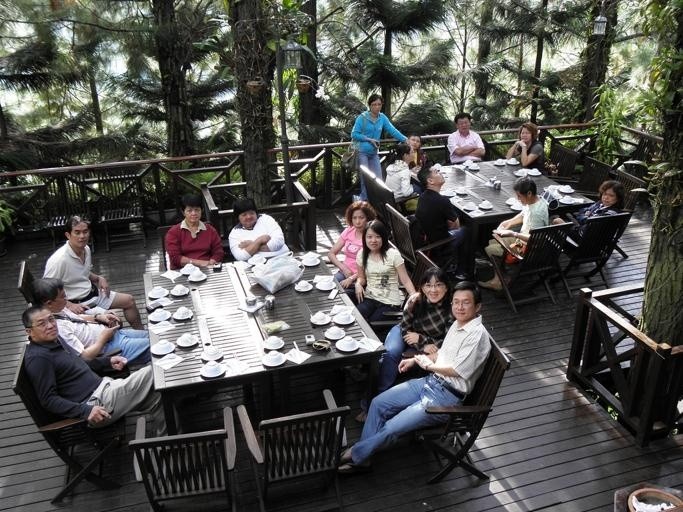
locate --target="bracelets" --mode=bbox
[514,231,518,237]
[348,277,354,281]
[425,363,433,370]
[96,275,103,282]
[94,312,101,321]
[190,258,192,264]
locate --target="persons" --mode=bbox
[350,93,548,203]
[354,219,417,321]
[327,199,378,306]
[413,165,481,280]
[355,266,457,423]
[21,306,217,457]
[475,177,549,293]
[164,192,225,271]
[228,197,290,263]
[332,279,492,474]
[41,215,145,329]
[30,277,152,367]
[550,179,625,263]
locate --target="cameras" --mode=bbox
[306,335,315,345]
[213,262,222,269]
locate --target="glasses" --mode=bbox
[25,317,54,328]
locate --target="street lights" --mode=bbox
[268,9,319,240]
[584,0,614,129]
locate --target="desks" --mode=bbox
[140,251,388,392]
[405,155,596,225]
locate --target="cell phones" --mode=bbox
[108,319,118,328]
[328,289,339,299]
[145,302,162,312]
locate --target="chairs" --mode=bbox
[560,156,612,198]
[385,202,465,279]
[354,164,396,225]
[13,261,36,299]
[366,253,419,333]
[563,211,634,292]
[543,143,579,181]
[583,170,645,215]
[488,220,574,312]
[130,406,238,511]
[409,328,514,488]
[10,340,134,508]
[236,389,356,511]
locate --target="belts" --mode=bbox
[432,372,467,403]
[67,280,98,303]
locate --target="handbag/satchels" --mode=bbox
[340,152,359,173]
[540,190,560,211]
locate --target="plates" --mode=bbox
[143,258,359,377]
[627,488,683,512]
[429,158,574,216]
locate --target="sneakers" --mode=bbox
[478,278,504,292]
